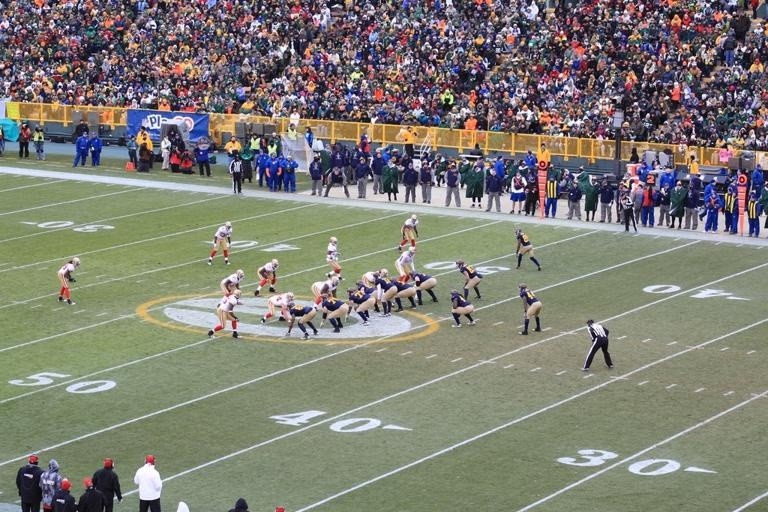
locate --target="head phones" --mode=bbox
[144,456,157,464]
[103,459,115,469]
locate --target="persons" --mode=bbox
[228,498,250,512]
[177,502,190,512]
[58,258,79,305]
[39,459,63,512]
[133,455,162,512]
[77,479,109,512]
[16,457,44,512]
[581,320,614,370]
[91,458,123,512]
[0,0,768,236]
[51,478,78,512]
[207,213,544,338]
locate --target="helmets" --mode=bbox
[236,270,244,279]
[409,246,416,255]
[287,292,294,300]
[411,214,417,222]
[450,289,458,296]
[356,280,363,288]
[331,276,340,286]
[234,290,242,298]
[225,221,232,230]
[72,258,80,267]
[347,287,356,296]
[390,278,398,284]
[373,268,387,278]
[329,236,337,246]
[410,271,417,279]
[455,259,464,268]
[519,283,527,290]
[272,259,278,268]
[320,293,329,299]
[515,228,521,235]
[287,302,295,310]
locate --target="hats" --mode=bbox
[586,319,594,324]
[104,458,114,468]
[275,506,285,512]
[60,479,72,491]
[145,455,156,465]
[83,477,93,487]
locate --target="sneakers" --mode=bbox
[518,331,529,335]
[395,308,403,313]
[58,296,66,302]
[225,260,231,265]
[207,259,213,266]
[417,302,423,305]
[232,333,243,339]
[255,291,262,297]
[398,247,402,253]
[311,331,319,335]
[261,316,267,323]
[360,321,369,326]
[299,333,311,340]
[474,295,481,299]
[516,265,521,269]
[325,273,331,279]
[366,316,370,321]
[580,366,590,371]
[410,305,416,309]
[608,363,614,368]
[208,329,216,339]
[433,299,438,302]
[538,266,542,271]
[338,276,345,281]
[269,286,278,292]
[66,298,76,305]
[279,316,286,321]
[451,323,462,328]
[331,325,343,333]
[531,328,541,331]
[467,320,475,326]
[379,312,391,317]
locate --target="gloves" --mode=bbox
[516,252,518,258]
[524,310,527,318]
[286,333,291,337]
[320,323,324,329]
[229,244,231,249]
[213,243,217,248]
